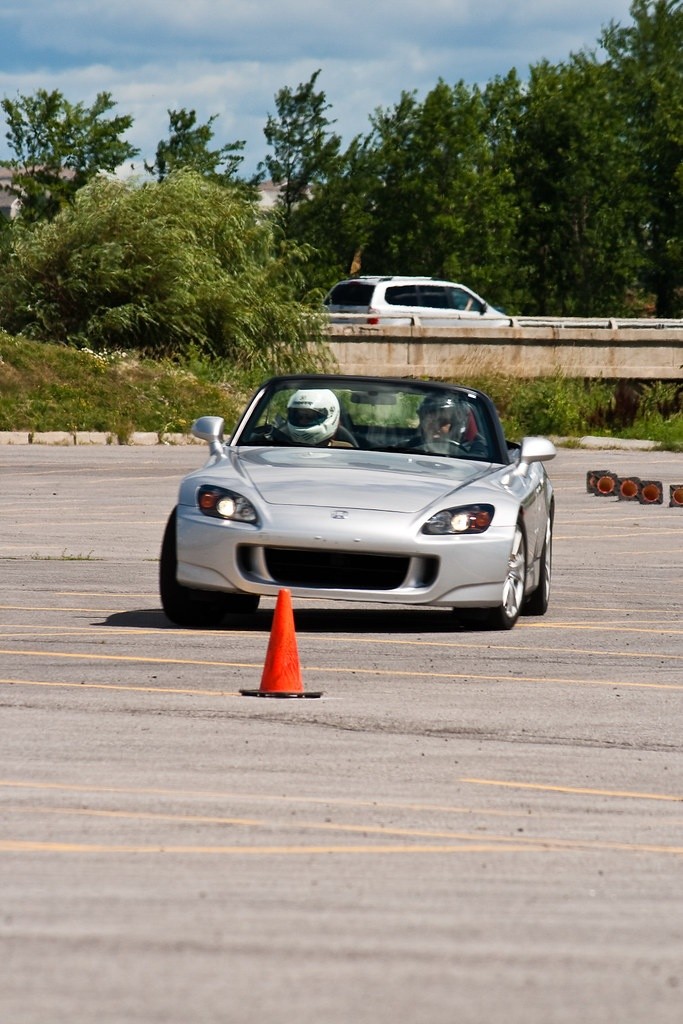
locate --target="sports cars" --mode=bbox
[158,373,559,631]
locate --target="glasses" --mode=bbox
[436,417,451,427]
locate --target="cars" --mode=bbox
[320,274,511,327]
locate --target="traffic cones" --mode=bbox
[668,484,683,508]
[637,480,664,505]
[614,476,641,502]
[593,473,618,496]
[586,469,612,493]
[237,588,325,699]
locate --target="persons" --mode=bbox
[275,389,354,448]
[464,297,474,311]
[398,393,487,456]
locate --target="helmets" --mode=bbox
[287,389,340,444]
[416,394,468,445]
